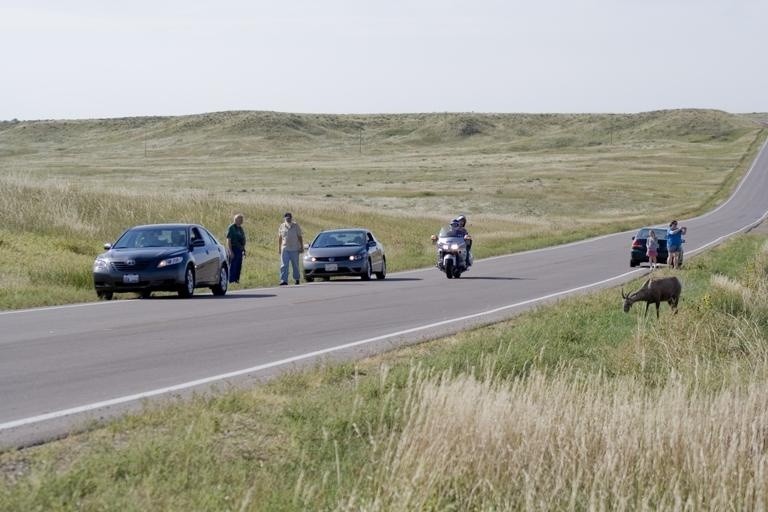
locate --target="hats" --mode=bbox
[284,213,291,219]
[450,215,466,227]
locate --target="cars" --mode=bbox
[630,228,685,267]
[303,228,386,283]
[93,223,231,299]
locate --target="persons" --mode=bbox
[437,219,472,270]
[456,216,467,229]
[226,213,246,283]
[646,230,658,270]
[279,213,304,286]
[667,220,686,272]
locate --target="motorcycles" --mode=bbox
[431,224,473,280]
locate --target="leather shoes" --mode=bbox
[279,281,288,285]
[294,280,300,284]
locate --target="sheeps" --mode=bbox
[621,276,682,320]
